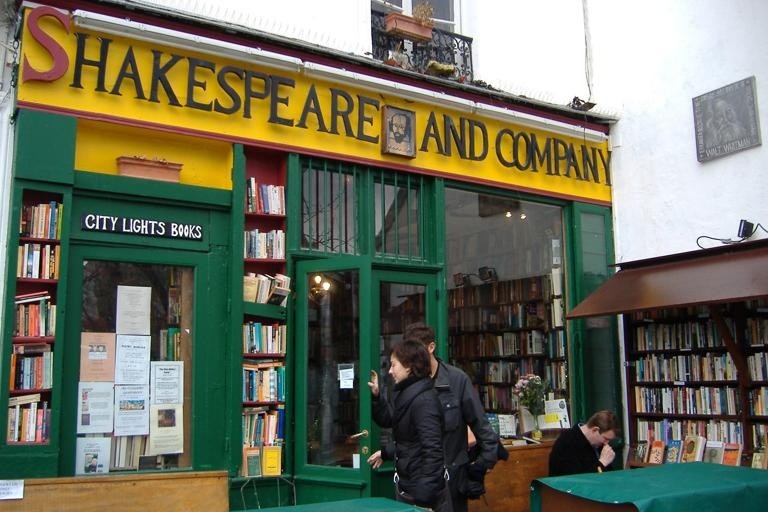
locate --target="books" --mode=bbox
[396,276,566,412]
[85,433,164,471]
[245,177,286,215]
[160,265,182,361]
[243,321,286,354]
[244,229,286,259]
[241,405,285,478]
[19,201,63,240]
[402,222,564,297]
[7,394,52,442]
[243,272,291,308]
[12,291,56,337]
[9,343,53,391]
[243,359,286,401]
[16,243,61,280]
[629,296,766,473]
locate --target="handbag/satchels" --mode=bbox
[392,463,450,511]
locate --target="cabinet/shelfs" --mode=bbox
[331,270,552,422]
[241,209,288,408]
[621,294,767,470]
[6,231,60,398]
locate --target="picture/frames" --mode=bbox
[381,103,418,159]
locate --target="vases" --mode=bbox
[115,155,185,184]
[383,10,435,45]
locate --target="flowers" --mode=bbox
[508,371,557,431]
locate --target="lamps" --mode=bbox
[309,272,336,303]
[694,218,768,249]
[478,266,498,284]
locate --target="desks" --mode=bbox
[218,493,435,512]
[527,461,767,512]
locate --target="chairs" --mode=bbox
[238,473,297,510]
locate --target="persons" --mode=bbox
[368,322,499,512]
[388,113,413,154]
[368,341,452,511]
[549,409,621,476]
